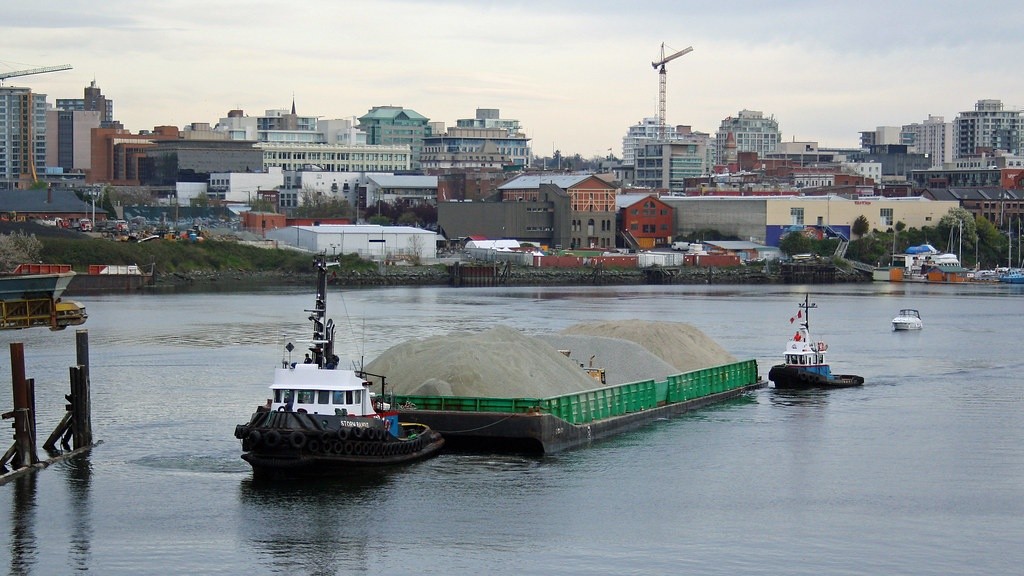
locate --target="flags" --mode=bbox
[790,310,801,324]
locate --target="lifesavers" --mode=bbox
[278,406,286,412]
[309,426,424,456]
[247,431,262,449]
[292,432,307,449]
[384,419,390,431]
[264,431,281,447]
[234,423,248,439]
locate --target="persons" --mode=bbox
[285,398,293,412]
[327,361,334,369]
[304,354,312,364]
[794,331,801,341]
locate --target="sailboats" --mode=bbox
[995,217,1024,283]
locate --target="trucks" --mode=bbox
[43,216,129,233]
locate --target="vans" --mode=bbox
[672,242,690,251]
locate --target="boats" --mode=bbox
[768,292,865,388]
[892,309,922,330]
[243,248,445,480]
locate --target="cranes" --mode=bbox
[0,63,74,80]
[651,42,694,141]
